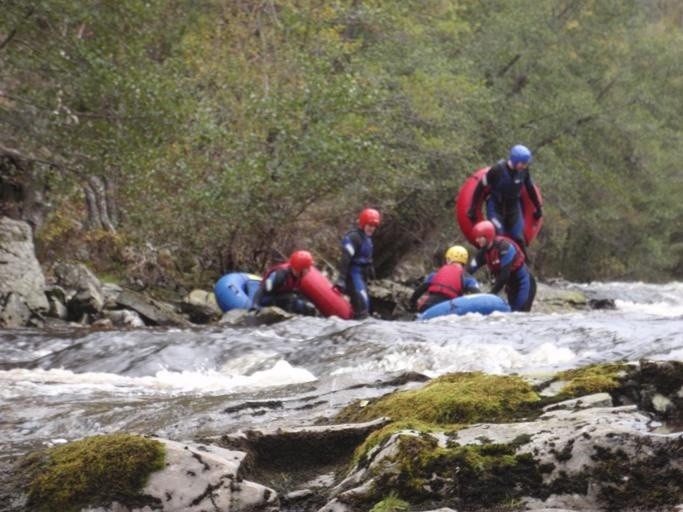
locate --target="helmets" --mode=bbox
[289,250,315,273]
[444,245,469,265]
[471,220,496,245]
[359,208,380,230]
[510,144,531,168]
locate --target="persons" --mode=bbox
[467,220,536,312]
[333,208,380,319]
[469,144,542,265]
[254,250,315,317]
[409,246,480,313]
